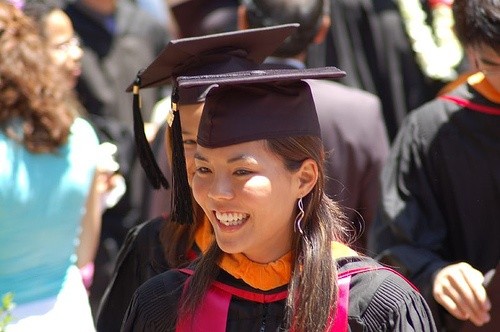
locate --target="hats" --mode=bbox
[125,23,301,187]
[177,66,346,150]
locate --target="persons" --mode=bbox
[373,0,500,332]
[0,0,107,332]
[119,67,437,332]
[97,23,303,332]
[13,0,461,211]
